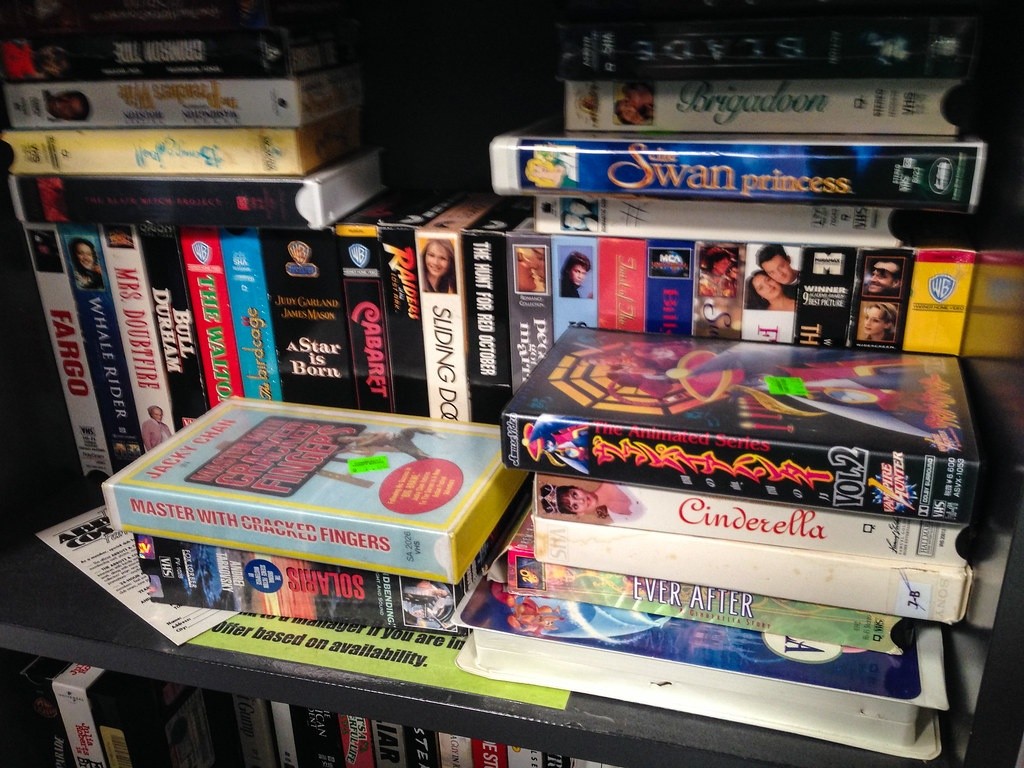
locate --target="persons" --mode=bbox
[530,248,547,290]
[420,241,456,294]
[621,83,655,118]
[559,211,599,232]
[742,271,796,313]
[697,250,736,298]
[863,254,901,297]
[69,237,105,290]
[43,89,90,123]
[568,199,600,219]
[28,230,64,273]
[559,251,592,298]
[142,406,171,450]
[333,427,445,461]
[615,98,642,127]
[860,304,898,343]
[537,482,645,528]
[756,244,800,300]
[515,249,536,292]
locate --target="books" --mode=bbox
[0,2,987,766]
